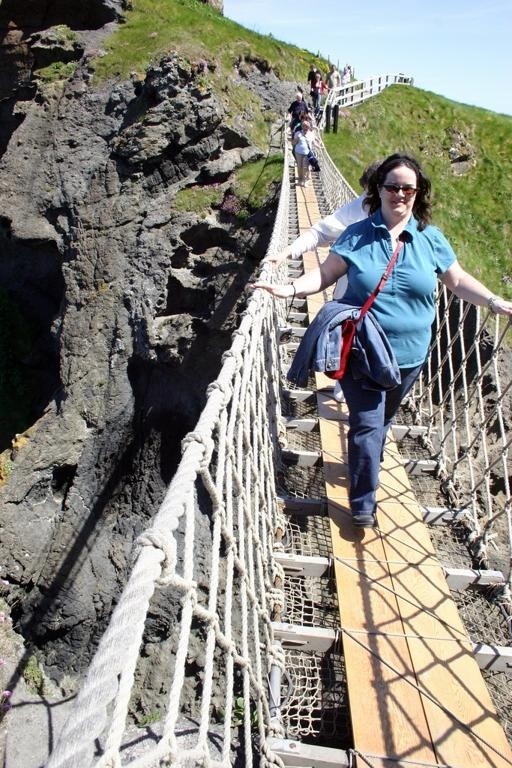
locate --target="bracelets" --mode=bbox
[488,297,504,312]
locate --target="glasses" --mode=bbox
[382,185,419,197]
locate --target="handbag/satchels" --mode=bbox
[324,319,355,379]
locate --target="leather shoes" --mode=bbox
[352,514,378,528]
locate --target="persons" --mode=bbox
[259,160,384,403]
[250,152,512,526]
[286,63,351,186]
